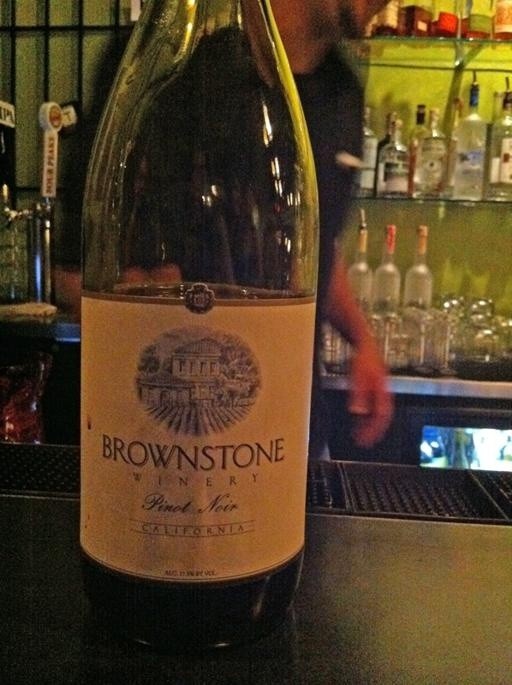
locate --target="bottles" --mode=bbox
[484,90,512,203]
[75,1,326,657]
[402,223,434,309]
[353,104,376,197]
[372,111,410,200]
[373,223,401,306]
[344,219,373,302]
[410,104,448,200]
[365,0,512,40]
[448,84,487,202]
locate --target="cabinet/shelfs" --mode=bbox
[349,34,512,211]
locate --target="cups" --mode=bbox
[0,302,59,446]
[320,292,512,374]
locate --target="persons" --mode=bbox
[36,0,396,509]
